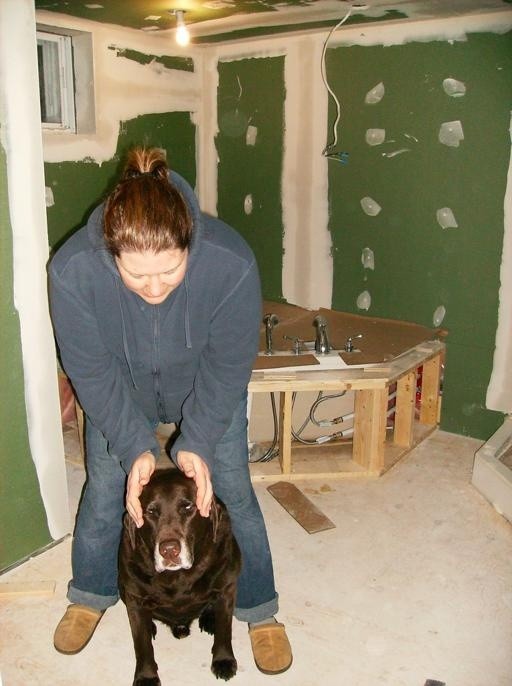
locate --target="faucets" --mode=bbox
[262,313,280,356]
[311,315,331,354]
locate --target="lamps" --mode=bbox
[174,9,193,48]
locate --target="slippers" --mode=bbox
[54,603,107,654]
[248,616,292,675]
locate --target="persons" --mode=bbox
[48,144,293,673]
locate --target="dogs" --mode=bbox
[115,466,242,686]
